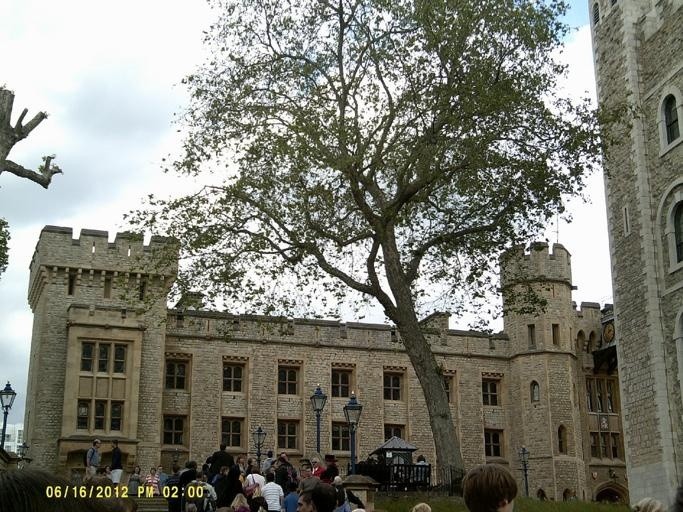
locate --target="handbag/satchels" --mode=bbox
[334,501,351,512]
[83,447,94,467]
[246,483,259,497]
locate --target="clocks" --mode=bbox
[603,323,615,342]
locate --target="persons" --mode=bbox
[460,462,517,512]
[665,479,682,512]
[367,458,374,464]
[0,463,103,512]
[376,455,384,464]
[78,476,138,512]
[408,454,429,483]
[630,496,668,512]
[411,502,431,512]
[86,438,366,512]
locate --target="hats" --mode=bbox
[323,455,338,462]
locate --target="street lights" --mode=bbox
[17,441,28,458]
[517,444,533,496]
[340,390,364,474]
[250,424,267,472]
[309,382,327,454]
[0,379,17,448]
